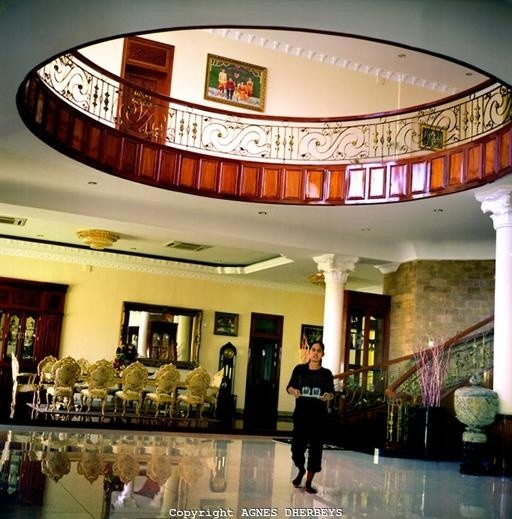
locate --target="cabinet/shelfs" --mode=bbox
[342,290,392,390]
[0,276,70,426]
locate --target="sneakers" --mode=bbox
[304,480,317,493]
[292,469,306,486]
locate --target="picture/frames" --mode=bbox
[299,324,323,351]
[204,52,268,112]
[418,123,447,152]
[214,311,239,336]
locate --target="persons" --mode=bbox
[285,340,337,494]
[218,67,256,102]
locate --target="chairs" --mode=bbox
[23,430,221,487]
[8,350,225,422]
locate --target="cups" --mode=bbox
[302,386,310,394]
[312,388,320,396]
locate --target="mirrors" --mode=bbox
[120,301,204,370]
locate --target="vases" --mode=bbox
[410,406,450,459]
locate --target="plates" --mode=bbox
[299,395,324,398]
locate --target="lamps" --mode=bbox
[77,228,120,250]
